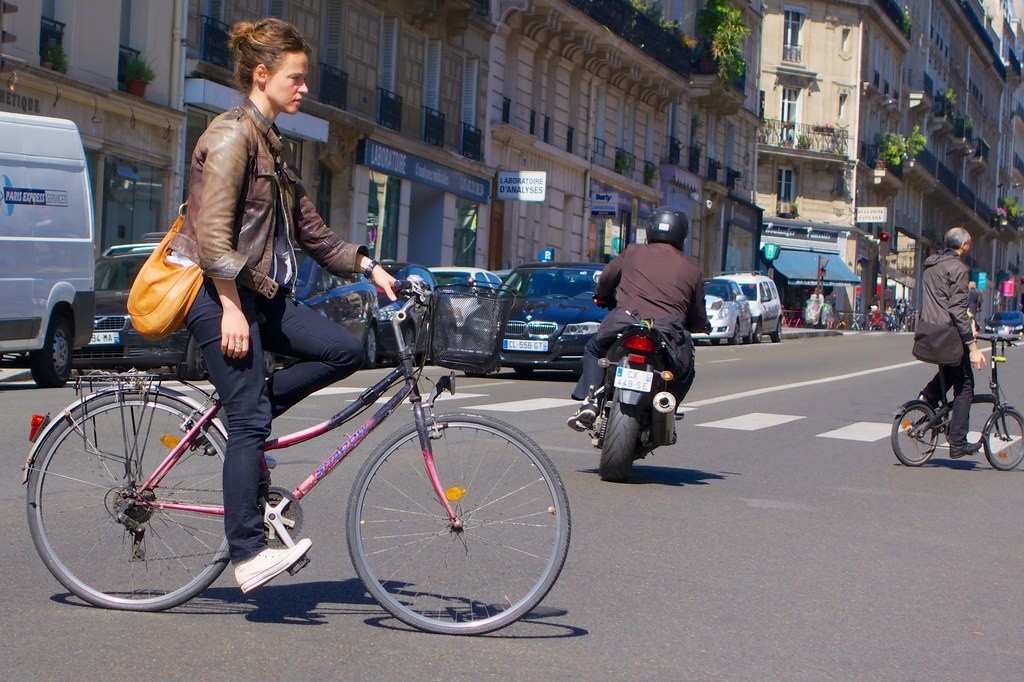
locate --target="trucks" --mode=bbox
[0,110,96,387]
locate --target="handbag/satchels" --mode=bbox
[912,320,965,366]
[596,307,694,383]
[126,215,205,340]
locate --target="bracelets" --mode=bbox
[364,259,382,279]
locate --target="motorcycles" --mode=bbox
[576,299,714,481]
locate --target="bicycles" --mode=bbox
[890,336,1024,470]
[21,274,571,635]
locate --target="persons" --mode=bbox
[967,281,982,333]
[805,287,825,302]
[169,18,398,594]
[917,228,987,459]
[568,207,707,432]
[869,305,881,325]
[885,299,906,333]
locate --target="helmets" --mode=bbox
[646,205,689,252]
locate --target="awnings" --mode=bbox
[772,250,861,286]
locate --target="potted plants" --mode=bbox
[759,120,811,149]
[42,45,69,74]
[789,202,798,211]
[124,58,155,98]
[875,132,909,168]
[694,0,752,81]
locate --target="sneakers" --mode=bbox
[917,391,939,409]
[949,442,982,459]
[263,452,276,468]
[234,538,313,594]
[673,432,677,444]
[566,403,600,432]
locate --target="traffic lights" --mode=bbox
[880,231,890,243]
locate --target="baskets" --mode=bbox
[430,283,515,374]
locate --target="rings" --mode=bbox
[236,342,241,348]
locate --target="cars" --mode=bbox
[68,232,609,380]
[690,271,783,344]
[984,310,1024,340]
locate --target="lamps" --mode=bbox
[9,71,17,91]
